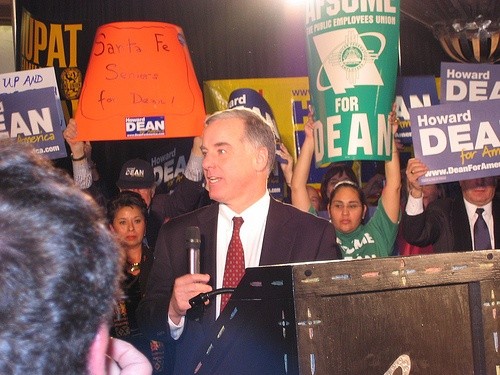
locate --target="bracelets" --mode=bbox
[69,152,86,161]
[88,160,97,169]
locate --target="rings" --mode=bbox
[394,118,399,123]
[411,170,415,174]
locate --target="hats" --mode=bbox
[114,156,155,190]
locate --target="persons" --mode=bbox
[138,110,341,375]
[404,157,500,253]
[64,119,202,260]
[108,191,170,375]
[84,141,107,193]
[0,137,152,375]
[291,102,402,259]
[274,142,447,256]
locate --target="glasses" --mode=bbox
[330,203,364,212]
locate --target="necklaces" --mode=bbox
[124,258,142,272]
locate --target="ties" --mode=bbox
[220,216,245,314]
[473,208,492,251]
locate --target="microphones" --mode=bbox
[185,226,201,283]
[189,288,236,308]
